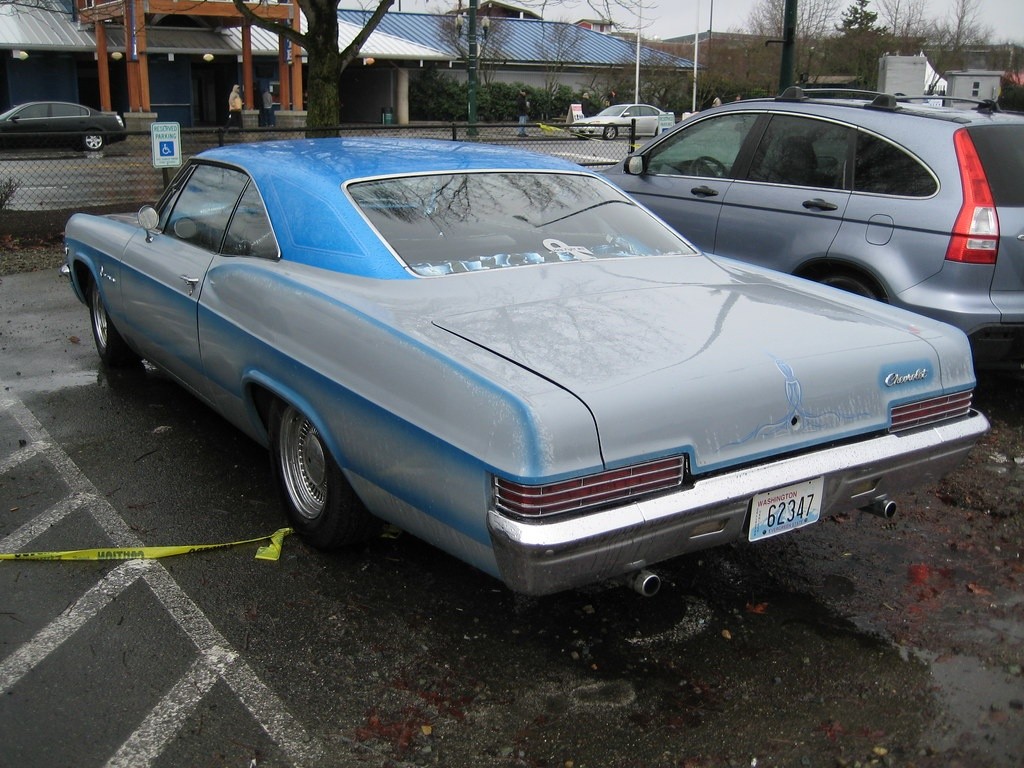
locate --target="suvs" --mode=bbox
[594,88,1024,354]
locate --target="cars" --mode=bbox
[61,132,992,600]
[570,103,674,141]
[0,102,126,152]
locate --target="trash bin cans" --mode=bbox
[382,107,393,125]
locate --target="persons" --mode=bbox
[583,93,590,116]
[735,94,741,100]
[518,92,529,137]
[712,97,721,108]
[224,84,243,128]
[610,90,617,105]
[263,89,274,126]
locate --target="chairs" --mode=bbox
[772,135,835,189]
[854,140,885,191]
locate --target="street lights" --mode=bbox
[455,14,490,135]
